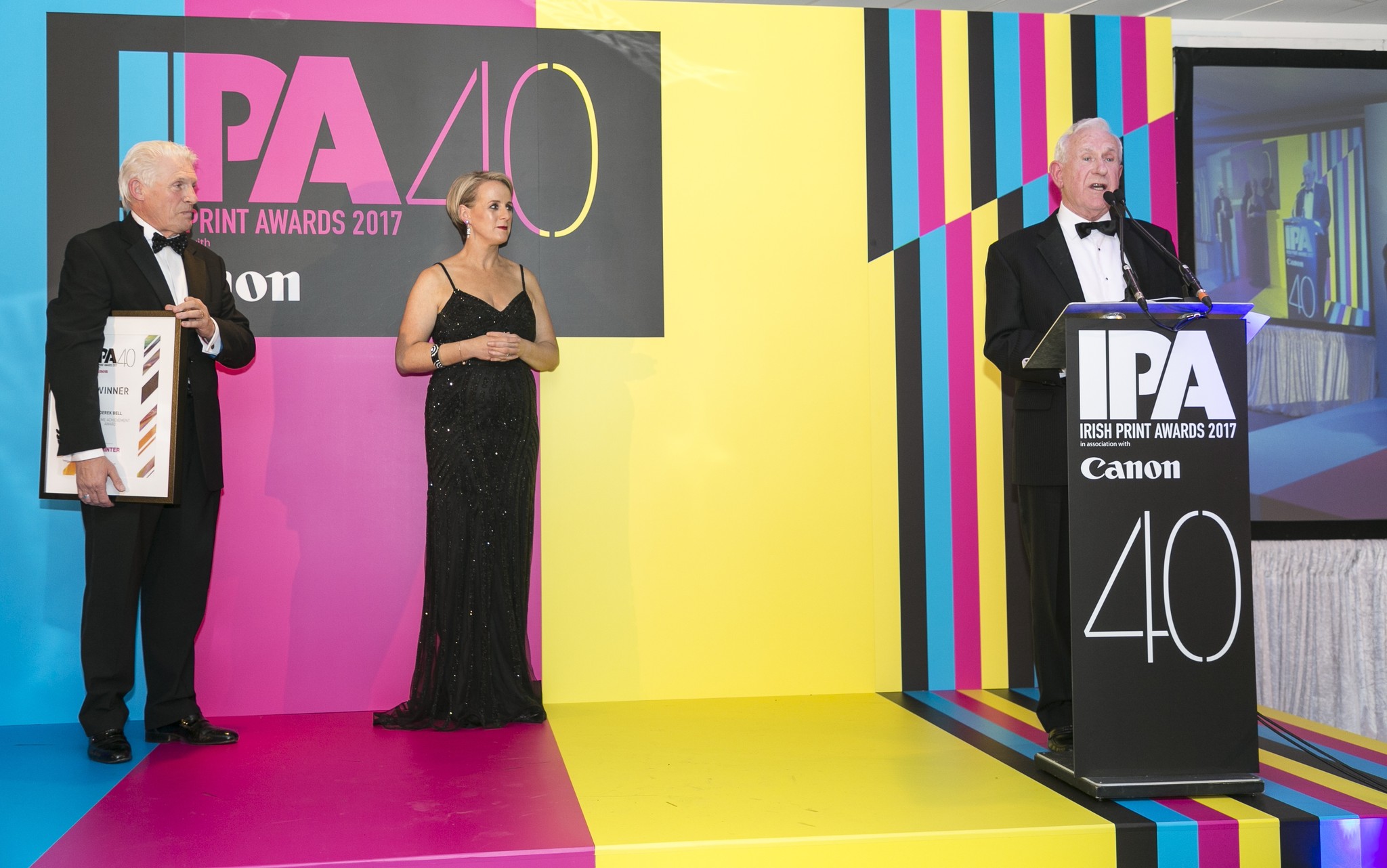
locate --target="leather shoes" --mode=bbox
[87,729,133,763]
[142,712,240,746]
[1046,726,1074,758]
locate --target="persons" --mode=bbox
[367,173,548,731]
[1294,159,1332,319]
[1213,181,1239,285]
[980,116,1200,757]
[46,140,258,765]
[1245,178,1268,286]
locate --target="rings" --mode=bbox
[507,353,511,358]
[83,495,89,499]
[199,309,204,319]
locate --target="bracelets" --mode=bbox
[430,342,446,369]
[457,340,468,365]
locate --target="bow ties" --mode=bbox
[1075,220,1117,239]
[152,232,188,255]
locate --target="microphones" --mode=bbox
[1103,188,1214,333]
[1292,183,1306,219]
